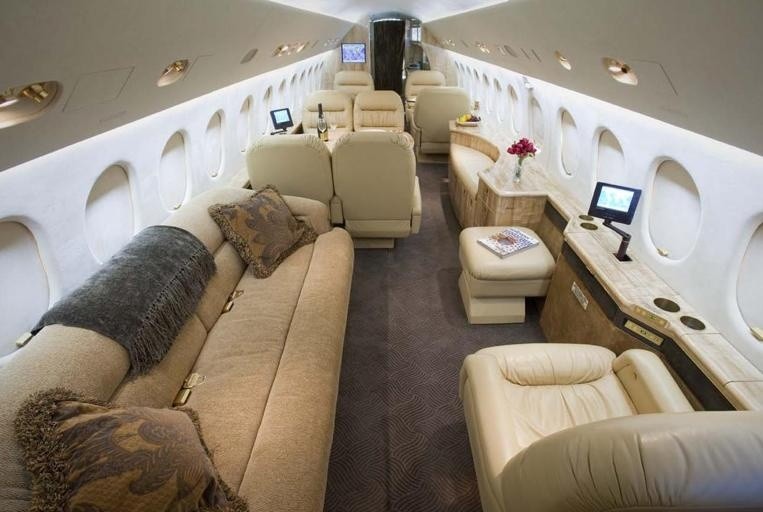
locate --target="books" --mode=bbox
[476,227,540,259]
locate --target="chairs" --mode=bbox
[243,133,334,229]
[352,90,405,131]
[402,69,446,123]
[300,89,353,134]
[331,130,423,249]
[407,86,474,164]
[332,70,375,96]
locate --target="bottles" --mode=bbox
[316,103,329,143]
[473,105,478,116]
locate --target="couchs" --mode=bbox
[0,183,356,511]
[457,342,763,512]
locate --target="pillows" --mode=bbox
[10,388,247,510]
[206,183,320,280]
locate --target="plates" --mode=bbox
[456,117,480,126]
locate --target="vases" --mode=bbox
[514,157,523,179]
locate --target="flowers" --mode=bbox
[506,138,537,158]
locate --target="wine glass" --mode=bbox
[317,118,326,143]
[328,115,340,138]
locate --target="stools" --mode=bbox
[454,224,557,326]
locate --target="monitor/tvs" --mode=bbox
[269,108,294,131]
[341,43,366,64]
[588,182,642,224]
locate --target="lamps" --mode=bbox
[409,16,421,43]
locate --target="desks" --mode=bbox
[300,127,415,156]
